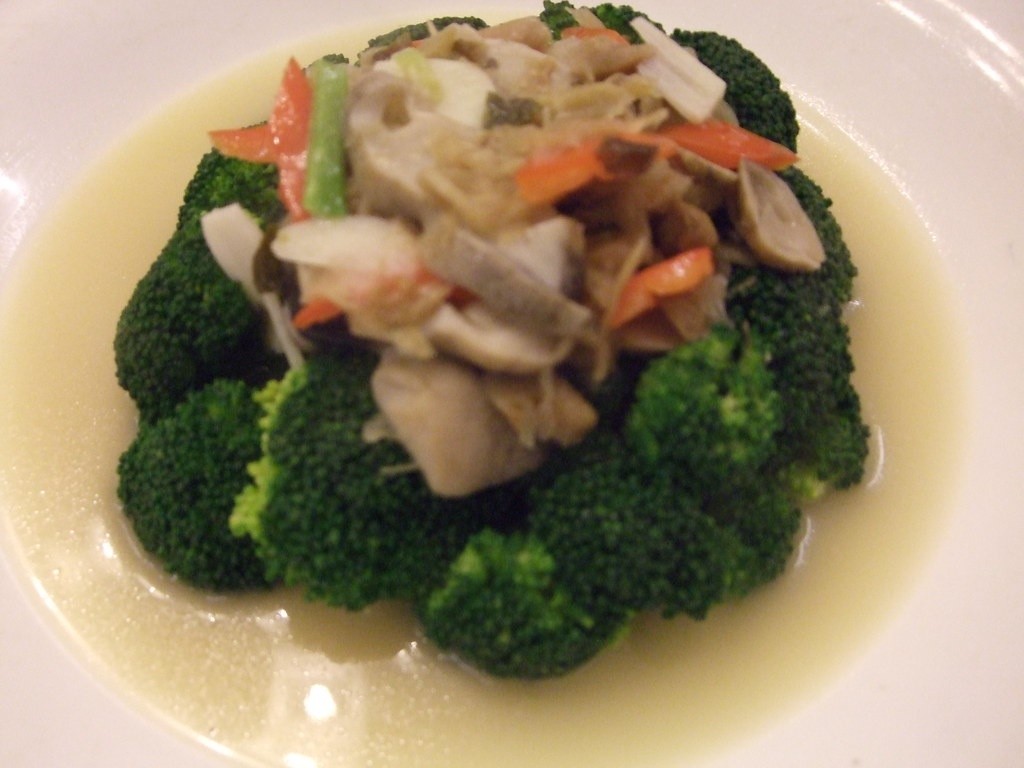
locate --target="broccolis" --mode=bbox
[113,0,872,684]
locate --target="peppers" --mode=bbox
[305,62,347,218]
